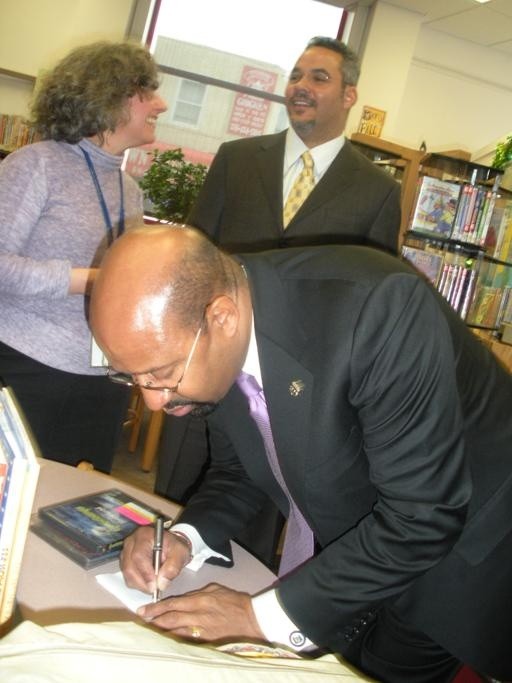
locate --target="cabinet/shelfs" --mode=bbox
[349,131,512,351]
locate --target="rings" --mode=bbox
[192,625,200,642]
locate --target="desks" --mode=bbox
[0,457,367,680]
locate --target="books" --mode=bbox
[40,484,172,550]
[1,385,41,626]
[32,513,127,570]
[400,173,511,349]
[0,111,49,152]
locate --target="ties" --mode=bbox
[282,152,317,231]
[234,371,315,580]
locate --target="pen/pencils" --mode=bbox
[153,515,163,601]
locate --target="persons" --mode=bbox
[144,33,400,574]
[83,219,510,683]
[1,39,171,480]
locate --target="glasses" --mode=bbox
[106,304,209,393]
